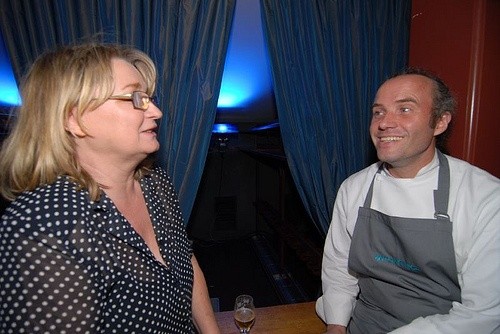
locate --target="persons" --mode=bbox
[315,65,500,334]
[0,32,222,334]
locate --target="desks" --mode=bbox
[213,301,328,334]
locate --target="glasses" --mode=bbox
[78,91,158,110]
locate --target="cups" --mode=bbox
[233,294,255,334]
